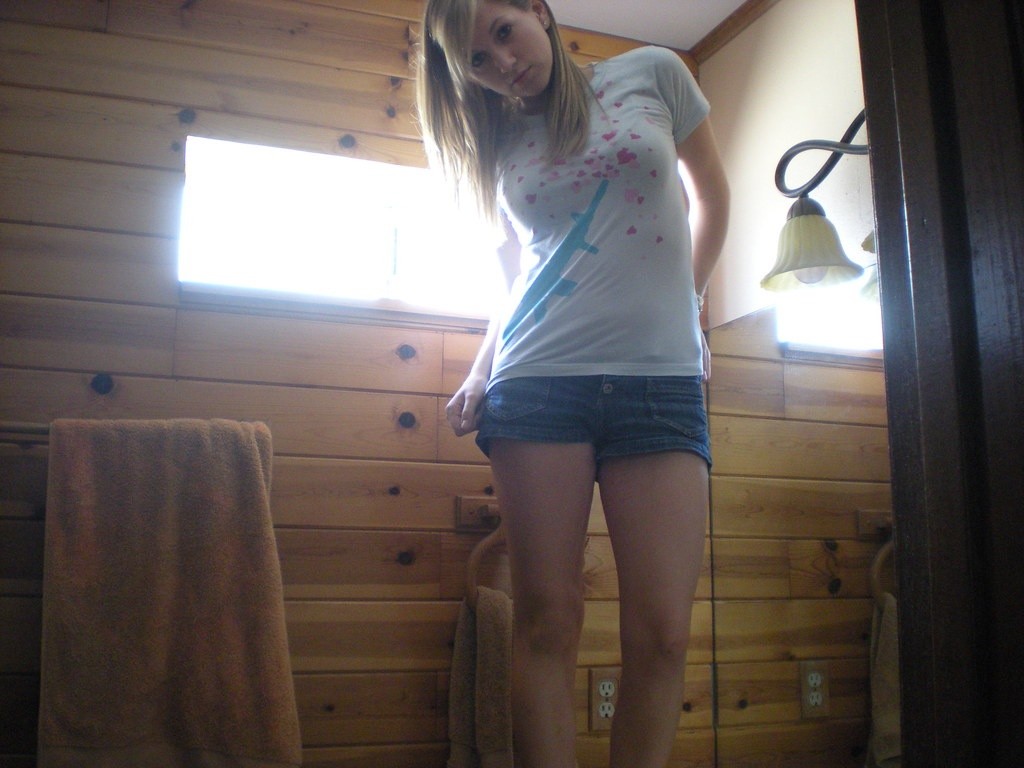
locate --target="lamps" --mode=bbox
[761,108,865,292]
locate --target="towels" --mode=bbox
[865,595,901,766]
[36,418,304,768]
[446,585,515,767]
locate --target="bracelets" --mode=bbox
[694,295,705,312]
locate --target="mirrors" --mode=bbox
[772,264,883,350]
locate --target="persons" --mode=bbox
[417,0,730,768]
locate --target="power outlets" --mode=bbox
[589,667,620,730]
[799,661,830,718]
